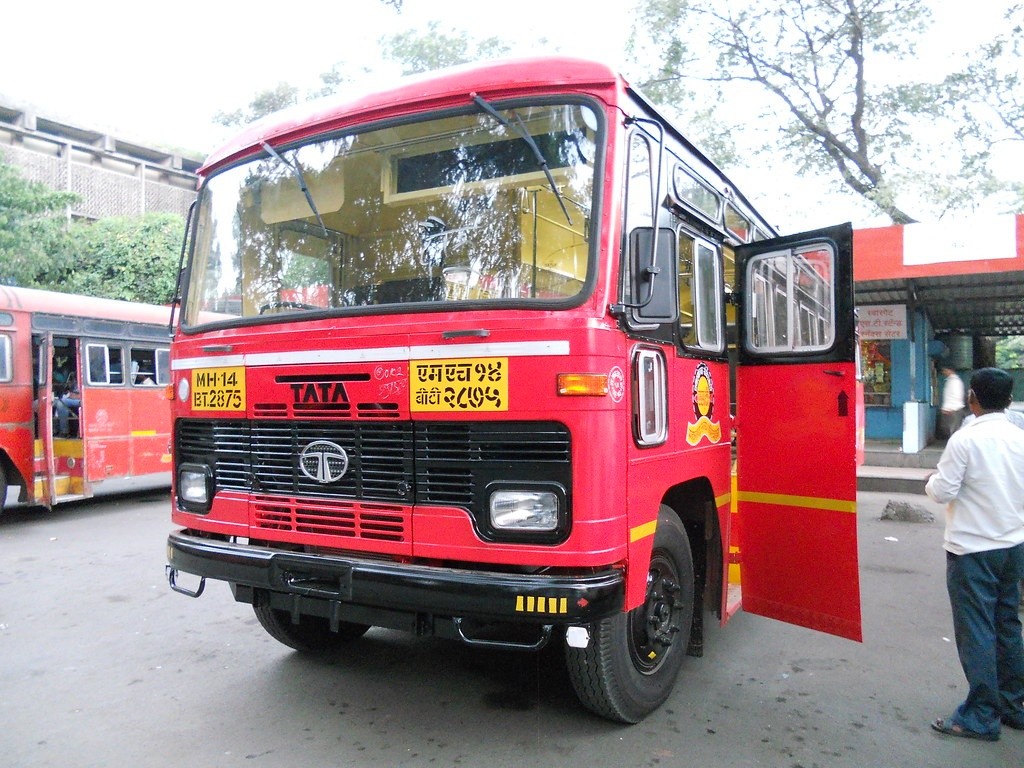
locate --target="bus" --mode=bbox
[165,56,860,724]
[0,286,241,516]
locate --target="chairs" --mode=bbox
[60,360,139,438]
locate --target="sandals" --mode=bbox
[931,717,998,741]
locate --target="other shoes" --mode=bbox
[54,432,71,438]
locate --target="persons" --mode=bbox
[139,366,155,384]
[33,357,99,438]
[923,368,1024,741]
[941,366,966,437]
